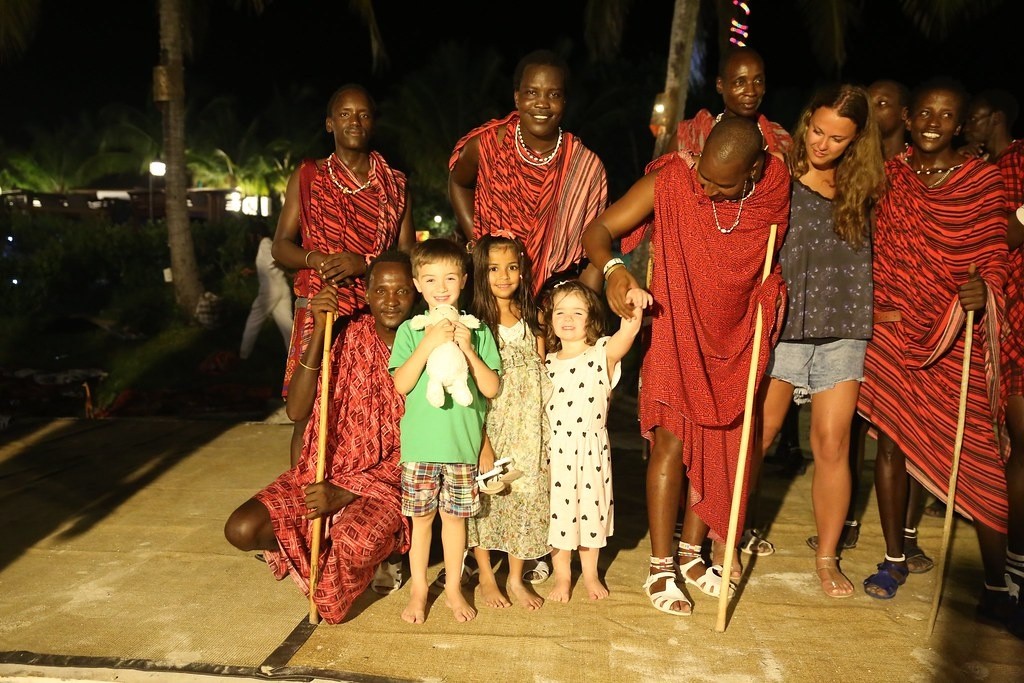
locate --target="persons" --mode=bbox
[239,221,295,359]
[223,247,420,625]
[470,230,554,611]
[388,237,505,625]
[962,86,1024,643]
[863,81,1011,599]
[543,280,654,605]
[582,116,791,616]
[445,57,607,585]
[836,78,934,574]
[676,52,795,557]
[711,85,890,599]
[269,82,416,473]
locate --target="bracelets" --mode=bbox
[306,250,315,268]
[602,257,625,274]
[299,360,322,371]
[605,263,624,281]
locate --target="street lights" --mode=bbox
[149,152,166,224]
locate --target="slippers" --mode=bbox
[371,561,403,595]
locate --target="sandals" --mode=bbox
[643,571,693,616]
[1005,550,1024,585]
[710,541,742,579]
[904,528,933,574]
[673,557,735,598]
[864,554,909,600]
[983,573,1024,639]
[522,559,550,584]
[807,519,858,551]
[495,457,521,486]
[815,555,854,597]
[475,468,505,495]
[436,550,481,588]
[739,530,774,556]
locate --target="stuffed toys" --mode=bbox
[410,301,480,410]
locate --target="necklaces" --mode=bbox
[515,119,562,166]
[723,174,755,202]
[711,178,747,234]
[327,152,376,196]
[927,162,966,190]
